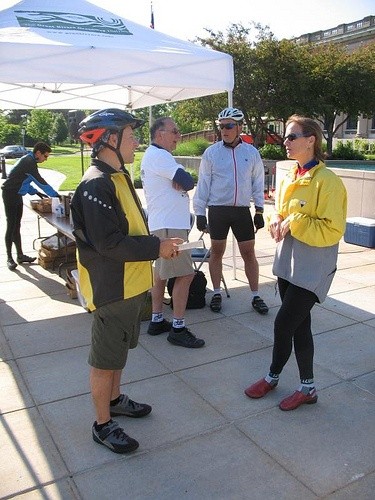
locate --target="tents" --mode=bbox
[0,0,235,182]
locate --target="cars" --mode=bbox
[0,144,31,158]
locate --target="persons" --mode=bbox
[194,106,269,314]
[140,117,205,348]
[1,141,61,269]
[70,108,184,453]
[244,116,347,411]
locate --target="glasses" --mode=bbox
[160,128,180,135]
[283,133,306,141]
[44,153,48,158]
[218,122,237,129]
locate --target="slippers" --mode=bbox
[279,390,318,411]
[244,376,272,399]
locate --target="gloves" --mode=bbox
[253,213,264,230]
[197,215,208,233]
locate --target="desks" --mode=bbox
[21,199,78,276]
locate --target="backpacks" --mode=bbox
[166,270,207,310]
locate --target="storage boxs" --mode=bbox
[344,216,375,248]
[29,199,52,213]
[38,232,79,299]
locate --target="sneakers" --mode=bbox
[110,394,152,418]
[167,327,205,348]
[6,259,18,269]
[91,420,139,454]
[147,318,173,335]
[251,295,269,314]
[210,293,223,312]
[17,254,37,265]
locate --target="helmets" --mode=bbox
[78,108,149,154]
[218,107,244,121]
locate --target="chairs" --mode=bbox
[190,231,231,298]
